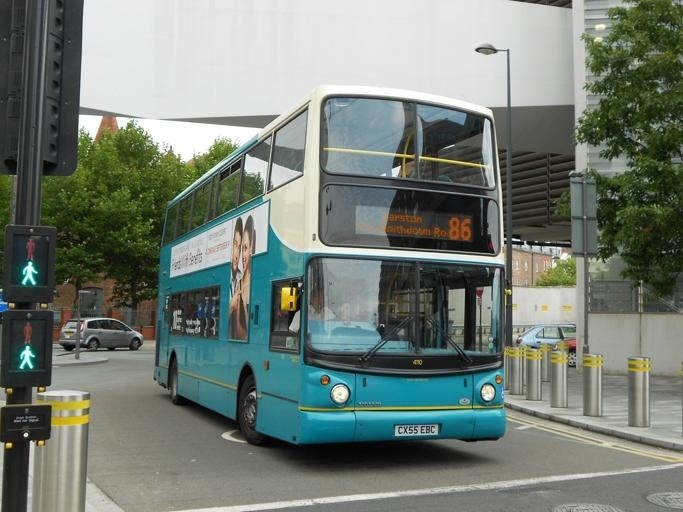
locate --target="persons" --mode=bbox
[288,287,338,336]
[235,215,256,340]
[227,217,242,338]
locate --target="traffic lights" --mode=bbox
[0,306,53,388]
[1,223,56,304]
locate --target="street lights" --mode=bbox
[472,40,514,349]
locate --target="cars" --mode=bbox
[60,318,143,354]
[516,325,577,369]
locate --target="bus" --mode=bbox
[149,84,508,451]
